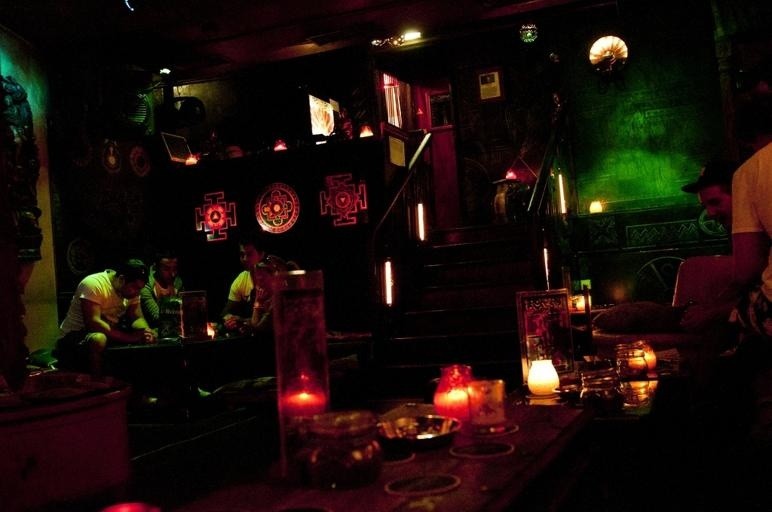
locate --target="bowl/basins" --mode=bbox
[375,415,463,452]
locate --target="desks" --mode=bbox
[99,334,252,422]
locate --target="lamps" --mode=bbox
[588,34,630,94]
[526,357,562,397]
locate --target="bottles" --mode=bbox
[300,409,380,486]
[528,340,657,415]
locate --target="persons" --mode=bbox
[221,235,301,333]
[140,250,184,340]
[673,63,770,512]
[51,258,159,379]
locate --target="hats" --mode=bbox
[682,159,737,192]
